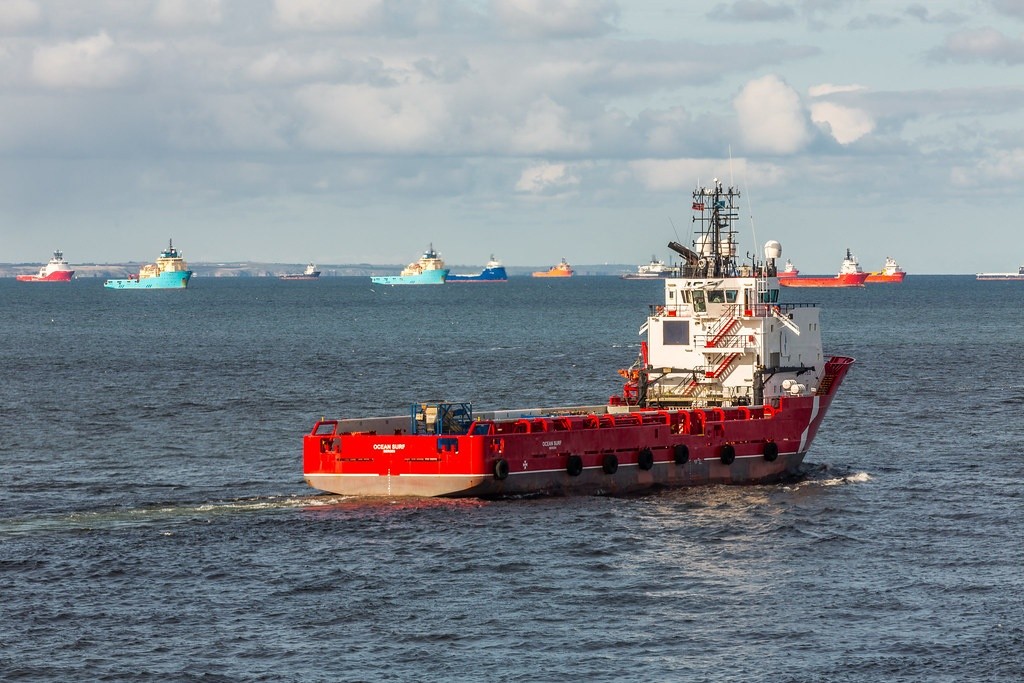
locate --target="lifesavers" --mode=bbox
[567,455,583,477]
[638,450,654,470]
[721,445,735,464]
[764,444,778,461]
[774,306,780,315]
[495,459,509,478]
[674,445,689,465]
[603,454,617,475]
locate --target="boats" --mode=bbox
[778,248,871,287]
[753,258,799,278]
[301,145,857,500]
[865,256,906,283]
[370,242,450,286]
[531,258,574,278]
[101,239,193,290]
[15,250,75,282]
[275,262,323,281]
[442,256,508,283]
[618,256,683,281]
